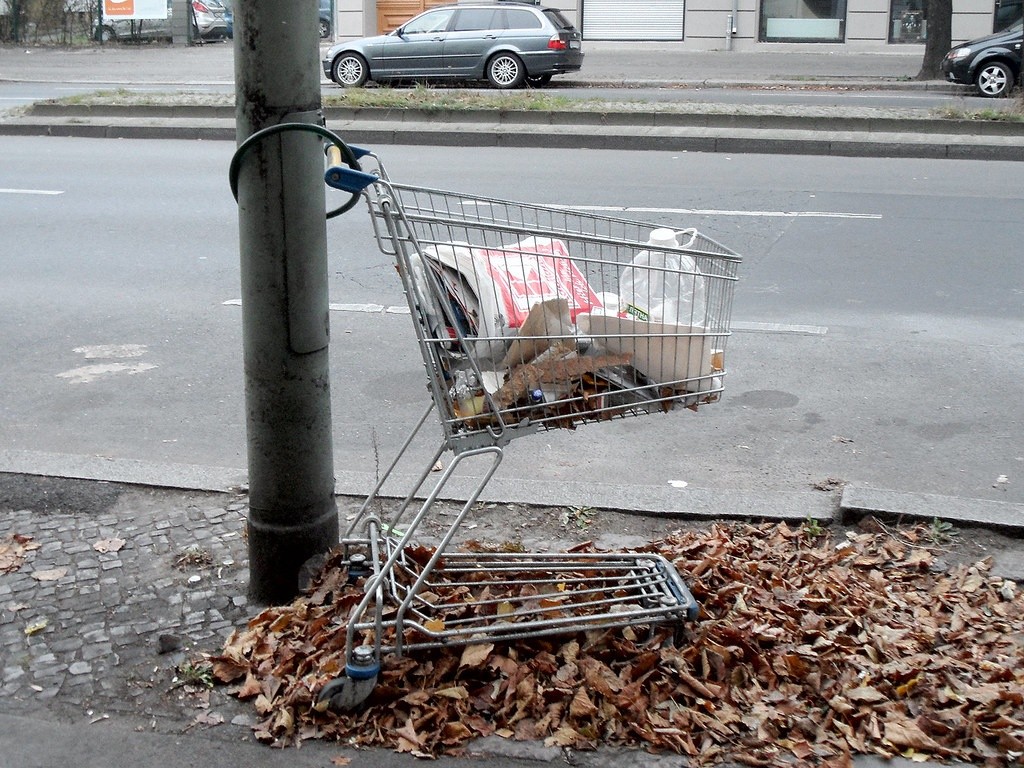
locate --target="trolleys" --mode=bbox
[316,134,745,722]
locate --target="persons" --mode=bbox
[903,14,921,34]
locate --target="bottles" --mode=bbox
[620,227,706,326]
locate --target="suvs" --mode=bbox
[321,0,585,89]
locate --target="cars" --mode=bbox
[88,0,234,45]
[939,15,1024,99]
[994,1,1024,32]
